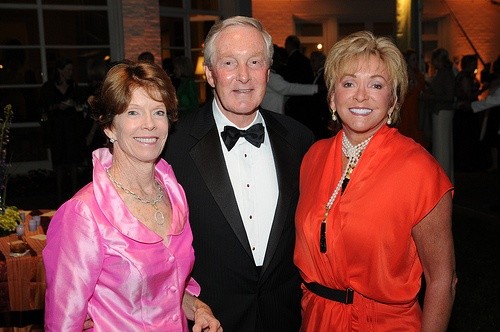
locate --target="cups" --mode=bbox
[16,224,25,235]
[32,216,40,226]
[28,220,37,232]
[21,213,25,222]
[9,234,26,253]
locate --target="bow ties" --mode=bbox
[221,122,265,151]
[315,73,318,77]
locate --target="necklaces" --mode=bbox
[102,165,169,226]
[319,131,374,252]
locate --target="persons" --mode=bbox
[298,29,457,332]
[37,35,500,248]
[43,62,225,332]
[165,15,316,332]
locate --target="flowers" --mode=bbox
[0,104,22,231]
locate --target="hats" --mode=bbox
[311,51,326,59]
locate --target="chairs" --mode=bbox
[0,255,47,332]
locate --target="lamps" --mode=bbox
[194,56,207,80]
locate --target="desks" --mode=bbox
[0,209,56,261]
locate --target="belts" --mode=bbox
[303,279,354,305]
